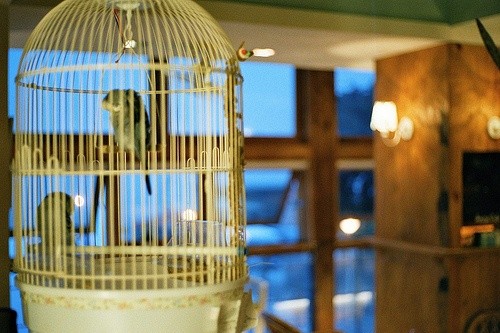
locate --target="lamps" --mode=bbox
[370,101,413,147]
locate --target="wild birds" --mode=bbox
[36,190,77,259]
[101,88,162,196]
